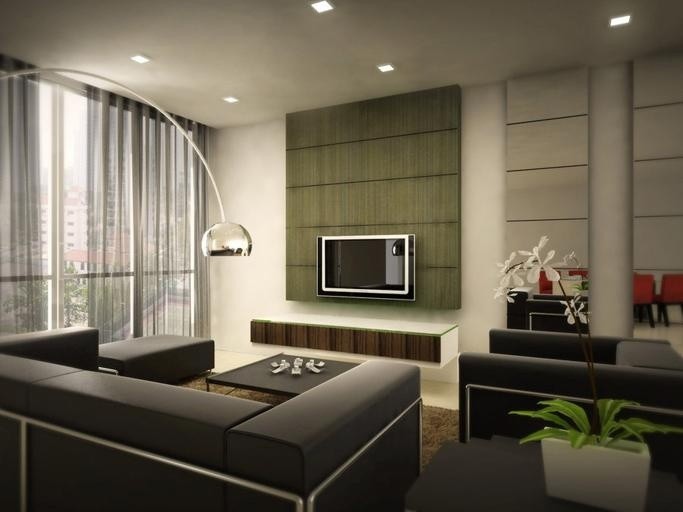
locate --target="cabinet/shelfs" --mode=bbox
[250,315,460,366]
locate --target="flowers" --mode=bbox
[493,225,683,449]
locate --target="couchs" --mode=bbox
[0,324,423,510]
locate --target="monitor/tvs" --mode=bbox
[316,234,415,301]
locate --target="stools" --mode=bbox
[98,334,215,393]
[403,439,683,512]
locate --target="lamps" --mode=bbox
[0,67,252,259]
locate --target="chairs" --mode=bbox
[456,326,683,442]
[537,266,683,328]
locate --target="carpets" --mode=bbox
[178,371,459,474]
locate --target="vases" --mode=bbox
[538,425,653,510]
